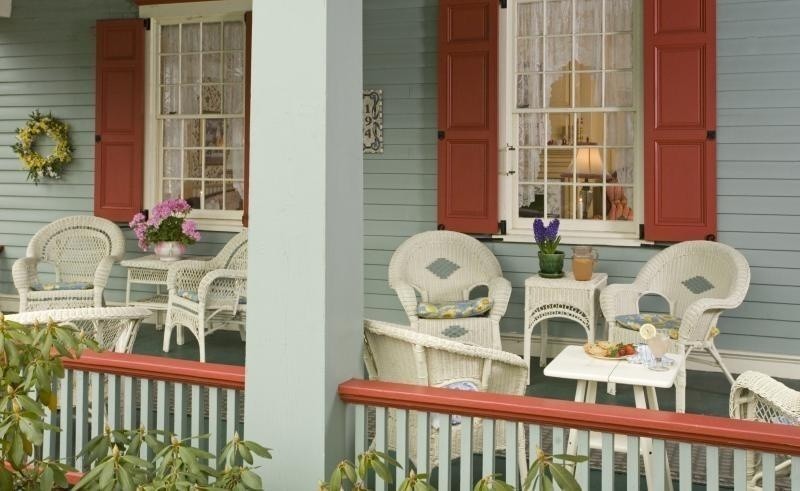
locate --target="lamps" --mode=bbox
[559,145,611,220]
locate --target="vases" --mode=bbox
[538,249,565,278]
[155,239,187,260]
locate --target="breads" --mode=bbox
[583,340,610,356]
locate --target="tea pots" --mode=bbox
[570,243,601,282]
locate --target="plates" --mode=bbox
[591,353,627,362]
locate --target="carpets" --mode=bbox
[121,376,800,491]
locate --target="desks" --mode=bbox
[522,270,607,386]
[544,344,682,477]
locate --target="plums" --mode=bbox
[615,345,633,356]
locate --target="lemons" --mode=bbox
[640,321,658,340]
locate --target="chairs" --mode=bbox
[12,215,125,312]
[162,229,249,363]
[364,230,527,352]
[729,367,800,491]
[5,306,152,354]
[600,239,751,414]
[364,319,528,491]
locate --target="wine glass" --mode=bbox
[645,332,673,372]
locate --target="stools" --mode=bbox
[120,254,209,345]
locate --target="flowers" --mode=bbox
[129,197,200,249]
[531,217,560,252]
[10,110,72,187]
